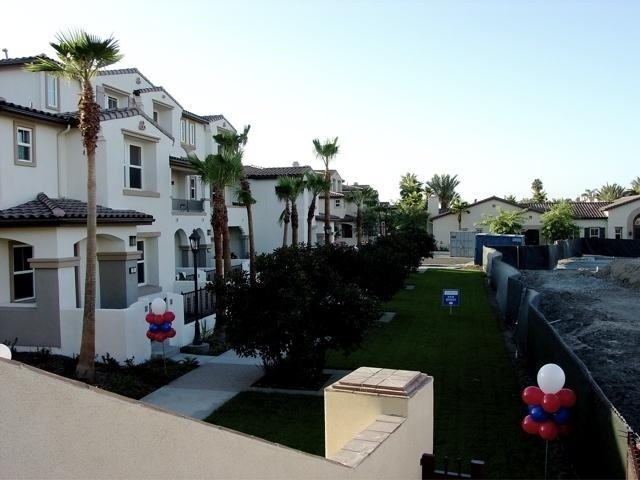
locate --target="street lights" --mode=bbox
[324,225,331,245]
[189,228,202,345]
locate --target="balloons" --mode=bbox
[144,296,178,343]
[517,363,580,442]
